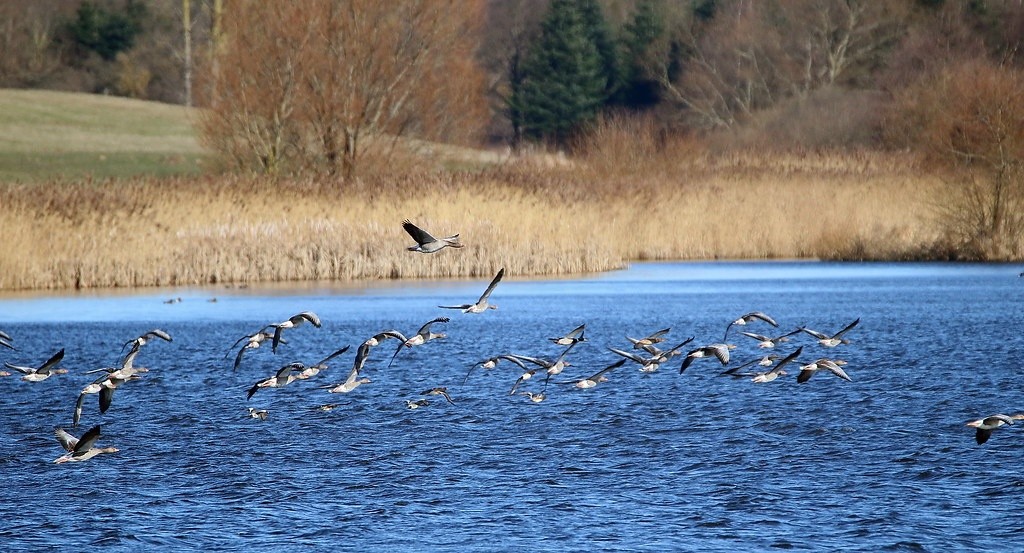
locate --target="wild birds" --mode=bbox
[164,297,216,304]
[223,269,865,427]
[73,327,177,428]
[0,330,70,382]
[964,414,1024,446]
[51,425,123,464]
[400,218,466,254]
